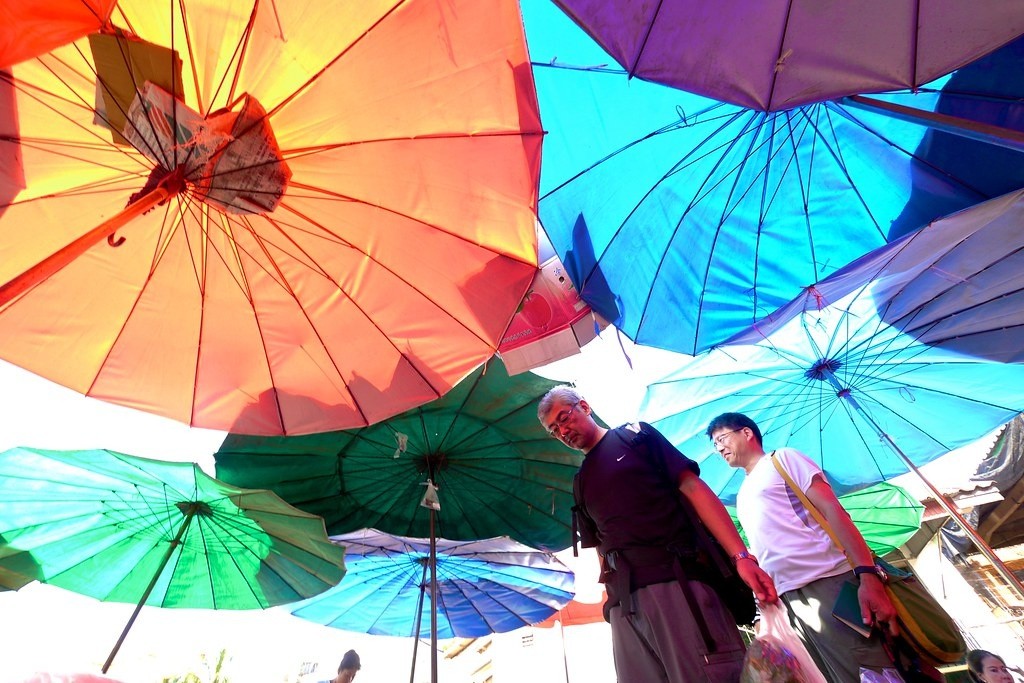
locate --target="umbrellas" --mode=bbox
[0,0,1024,683]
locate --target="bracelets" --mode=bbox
[730,552,759,569]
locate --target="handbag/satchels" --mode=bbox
[740,598,828,682]
[883,572,966,663]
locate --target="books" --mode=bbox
[831,580,874,639]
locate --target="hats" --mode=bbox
[338,649,360,673]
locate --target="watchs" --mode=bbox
[853,565,888,582]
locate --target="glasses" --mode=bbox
[549,402,578,439]
[714,428,743,448]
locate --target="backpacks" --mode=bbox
[571,425,756,628]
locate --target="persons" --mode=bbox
[752,591,761,634]
[705,413,905,683]
[537,385,779,683]
[968,650,1014,683]
[318,650,361,683]
